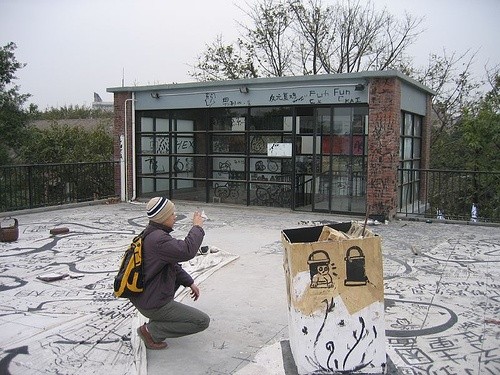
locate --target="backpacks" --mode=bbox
[114,227,163,299]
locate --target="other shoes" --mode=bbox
[137,322,167,349]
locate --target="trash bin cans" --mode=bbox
[280,222,388,375]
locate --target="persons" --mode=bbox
[127,196,210,350]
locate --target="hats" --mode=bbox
[146,196,175,224]
